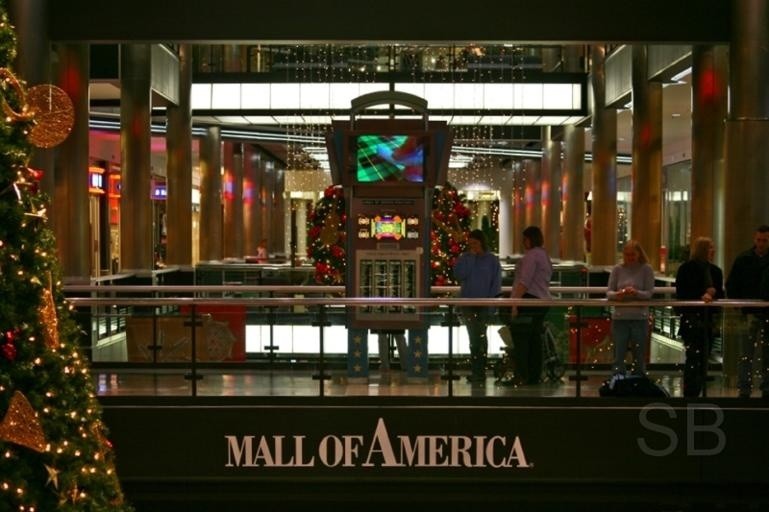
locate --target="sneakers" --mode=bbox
[501,376,526,385]
[467,374,485,381]
[738,388,751,396]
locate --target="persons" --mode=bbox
[607,241,655,374]
[725,225,768,399]
[501,226,553,387]
[673,235,722,400]
[449,230,502,381]
[155,234,166,265]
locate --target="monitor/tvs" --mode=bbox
[355,134,428,185]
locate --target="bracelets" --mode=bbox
[621,289,626,296]
